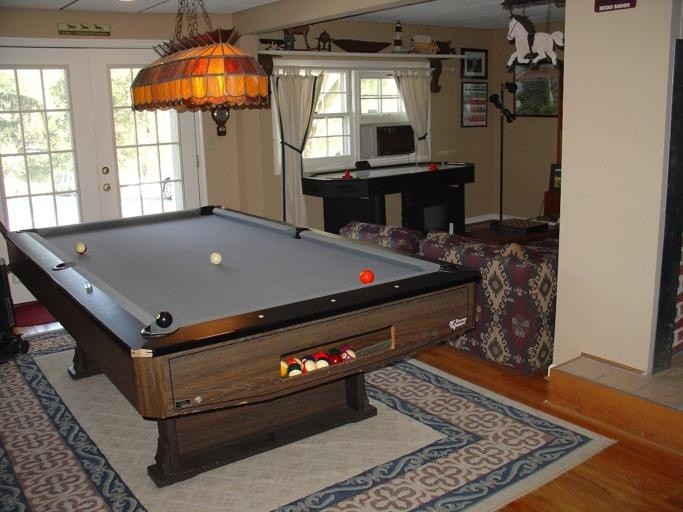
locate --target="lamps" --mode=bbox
[129,1,271,112]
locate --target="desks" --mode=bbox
[472,228,555,245]
[306,162,475,234]
[0,204,480,487]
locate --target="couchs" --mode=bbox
[340,221,559,376]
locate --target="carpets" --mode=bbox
[0,327,618,512]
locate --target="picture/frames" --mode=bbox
[461,48,487,128]
[513,59,563,118]
[549,163,561,193]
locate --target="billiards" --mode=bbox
[75,242,87,254]
[359,270,374,284]
[210,252,222,264]
[156,311,173,328]
[280,345,356,377]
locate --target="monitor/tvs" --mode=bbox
[359,122,417,159]
